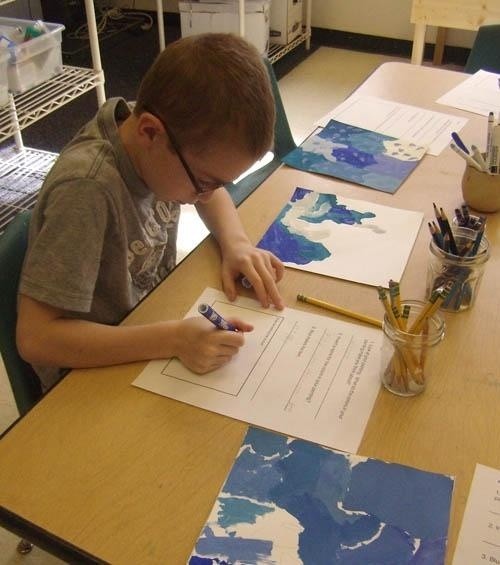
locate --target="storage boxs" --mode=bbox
[179,0,270,59]
[269,0,304,44]
[0,16,65,93]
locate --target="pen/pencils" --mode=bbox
[198,303,238,331]
[378,279,453,395]
[426,204,487,311]
[450,112,500,173]
[297,294,383,328]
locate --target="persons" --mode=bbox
[14,32,284,395]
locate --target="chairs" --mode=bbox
[0,208,44,557]
[462,24,500,74]
[222,59,294,198]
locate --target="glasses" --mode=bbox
[150,111,225,195]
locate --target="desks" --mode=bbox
[408,0,500,65]
[0,62,500,565]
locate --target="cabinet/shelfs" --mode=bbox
[0,0,109,232]
